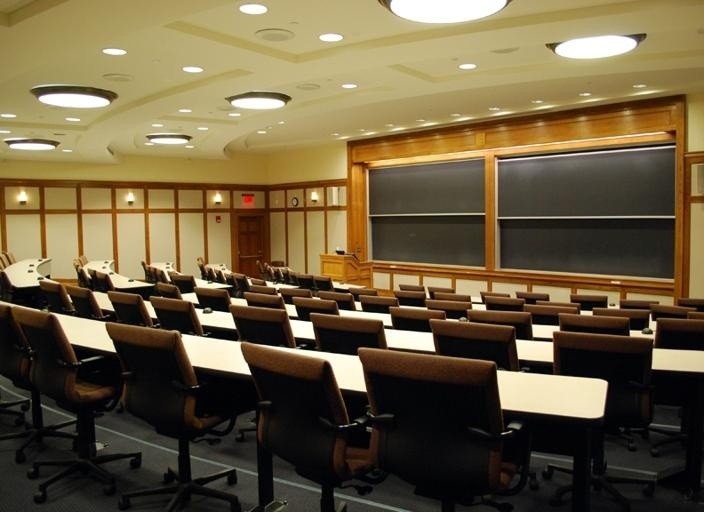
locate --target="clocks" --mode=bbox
[291,196,299,207]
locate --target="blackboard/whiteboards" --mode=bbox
[494,219,675,283]
[494,145,676,219]
[365,158,486,217]
[367,216,486,271]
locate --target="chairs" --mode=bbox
[0,251,703,512]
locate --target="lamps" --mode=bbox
[16,190,28,208]
[126,193,134,206]
[226,89,291,111]
[2,135,62,152]
[143,131,192,147]
[548,33,642,60]
[213,193,222,205]
[310,190,318,203]
[29,84,121,112]
[376,0,522,27]
